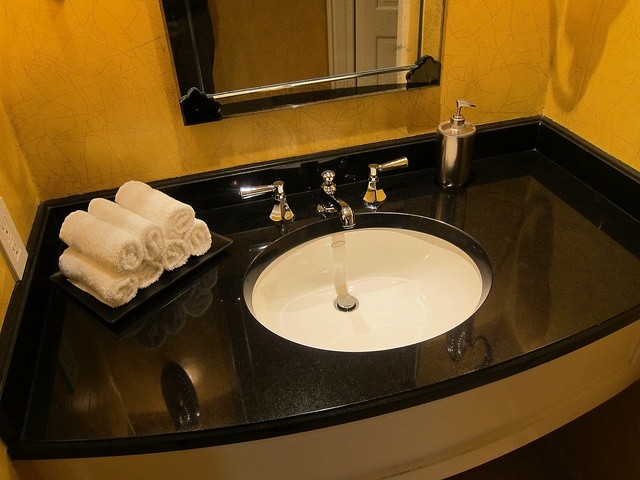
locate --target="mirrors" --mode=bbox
[159,0,448,127]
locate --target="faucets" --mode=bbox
[317,170,356,229]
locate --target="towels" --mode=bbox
[59,209,143,276]
[160,238,191,272]
[129,261,165,289]
[87,197,165,263]
[115,180,196,240]
[183,218,212,257]
[58,247,138,308]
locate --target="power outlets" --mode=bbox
[0,196,30,281]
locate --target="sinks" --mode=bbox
[243,212,494,353]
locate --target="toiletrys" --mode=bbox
[436,100,476,192]
